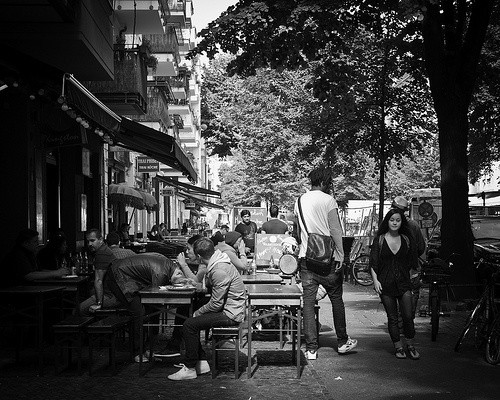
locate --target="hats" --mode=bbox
[225,232,241,245]
[393,196,408,210]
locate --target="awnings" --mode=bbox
[115,116,197,181]
[162,177,225,210]
[109,184,158,210]
[47,72,120,142]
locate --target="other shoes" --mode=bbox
[154,347,181,357]
[400,333,405,336]
[135,351,161,362]
[173,362,185,367]
[392,344,407,359]
[406,344,420,360]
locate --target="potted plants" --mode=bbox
[191,223,198,236]
[179,63,191,77]
[141,37,156,60]
[173,114,184,129]
[144,56,159,72]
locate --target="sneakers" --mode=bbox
[168,364,198,380]
[304,350,317,359]
[196,360,210,374]
[337,336,357,353]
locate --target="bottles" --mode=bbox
[61,250,89,276]
[269,255,274,268]
[251,255,257,275]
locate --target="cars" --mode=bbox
[428,214,500,287]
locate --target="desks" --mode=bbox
[0,234,304,380]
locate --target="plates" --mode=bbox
[167,285,196,290]
[266,269,281,274]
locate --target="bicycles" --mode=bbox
[453,255,500,365]
[417,250,458,342]
[353,244,426,286]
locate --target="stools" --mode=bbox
[42,317,94,376]
[87,317,133,375]
[211,326,241,380]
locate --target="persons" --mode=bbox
[261,205,292,235]
[236,210,258,253]
[391,196,426,337]
[369,209,420,360]
[293,167,358,360]
[10,222,248,381]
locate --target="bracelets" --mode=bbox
[96,301,102,305]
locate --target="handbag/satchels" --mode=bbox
[306,233,335,268]
